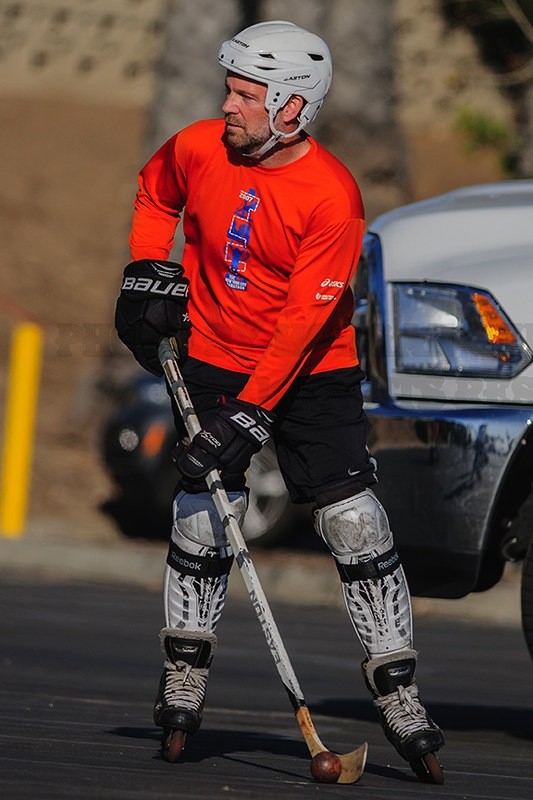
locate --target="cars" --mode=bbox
[92,297,348,555]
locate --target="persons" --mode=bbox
[112,20,445,783]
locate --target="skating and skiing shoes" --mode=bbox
[153,624,216,759]
[362,649,445,783]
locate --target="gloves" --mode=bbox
[114,259,190,378]
[174,394,278,490]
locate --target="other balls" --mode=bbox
[311,751,342,783]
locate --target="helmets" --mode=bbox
[217,21,331,140]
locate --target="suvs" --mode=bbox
[348,180,532,658]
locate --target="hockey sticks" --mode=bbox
[154,335,369,784]
